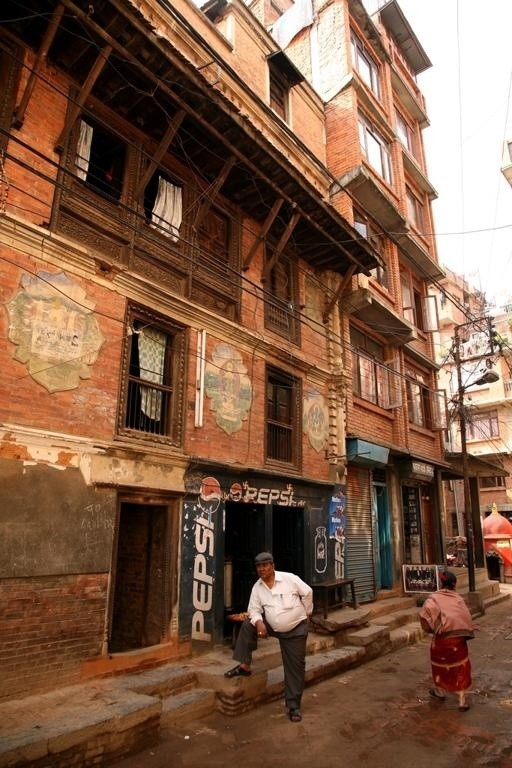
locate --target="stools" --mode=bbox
[227,612,257,651]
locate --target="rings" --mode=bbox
[258,631,263,636]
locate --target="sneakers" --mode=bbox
[428,688,471,712]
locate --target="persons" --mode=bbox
[223,551,314,723]
[419,570,476,711]
[405,566,435,588]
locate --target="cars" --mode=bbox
[447,554,463,567]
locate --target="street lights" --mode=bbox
[452,370,499,592]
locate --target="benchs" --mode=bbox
[310,579,359,620]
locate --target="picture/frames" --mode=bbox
[401,564,440,594]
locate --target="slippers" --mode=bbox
[289,708,302,722]
[224,664,252,678]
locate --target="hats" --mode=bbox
[254,552,274,566]
